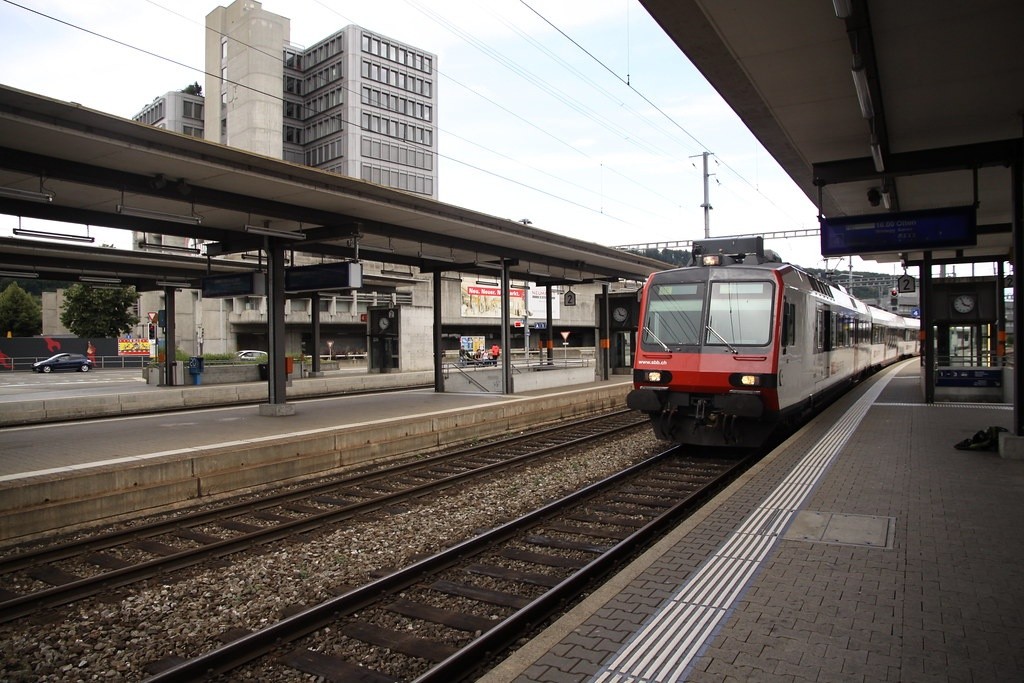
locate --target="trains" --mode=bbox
[625,235,986,449]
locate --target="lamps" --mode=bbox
[138,227,200,254]
[78,260,122,283]
[380,262,413,276]
[115,171,202,225]
[13,214,95,242]
[0,248,40,279]
[347,232,395,254]
[474,251,504,270]
[0,163,56,205]
[511,278,531,289]
[240,234,291,266]
[244,197,305,239]
[156,267,191,287]
[476,273,498,286]
[418,241,454,263]
[612,307,628,323]
[440,270,463,283]
[527,259,551,277]
[592,273,609,285]
[563,267,583,282]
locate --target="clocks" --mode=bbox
[954,295,975,313]
[379,317,389,329]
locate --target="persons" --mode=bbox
[87,341,97,367]
[459,343,500,367]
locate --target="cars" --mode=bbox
[234,350,268,360]
[31,352,93,374]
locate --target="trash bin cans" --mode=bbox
[950,355,963,367]
[255,364,269,380]
[171,360,184,385]
